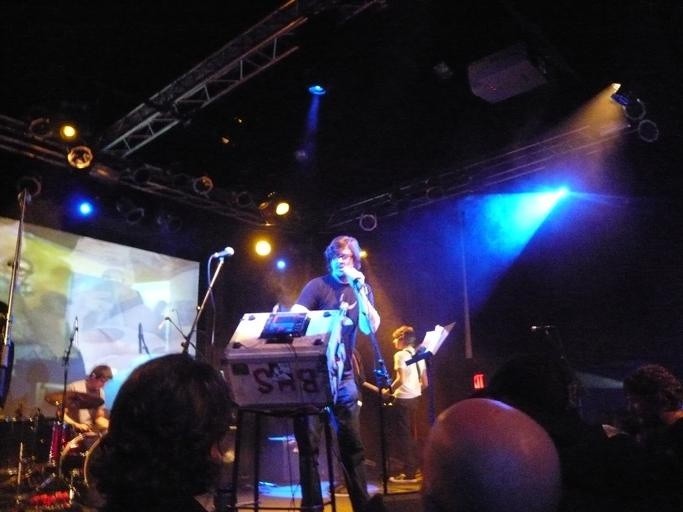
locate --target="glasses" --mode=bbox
[331,254,354,260]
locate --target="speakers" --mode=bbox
[366,491,423,512]
[268,436,301,485]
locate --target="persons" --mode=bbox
[601,364,682,511]
[51,365,113,461]
[379,324,430,484]
[418,397,564,511]
[82,354,234,512]
[286,235,384,512]
[332,345,388,494]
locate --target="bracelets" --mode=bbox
[388,385,393,394]
[378,386,383,398]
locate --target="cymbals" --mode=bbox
[43,391,104,409]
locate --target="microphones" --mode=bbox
[213,247,236,259]
[18,191,33,203]
[74,316,80,347]
[139,323,143,354]
[531,325,555,332]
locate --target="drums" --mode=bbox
[57,428,116,508]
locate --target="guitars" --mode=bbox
[363,381,395,408]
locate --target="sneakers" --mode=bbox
[357,493,385,512]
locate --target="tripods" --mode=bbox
[13,355,88,506]
